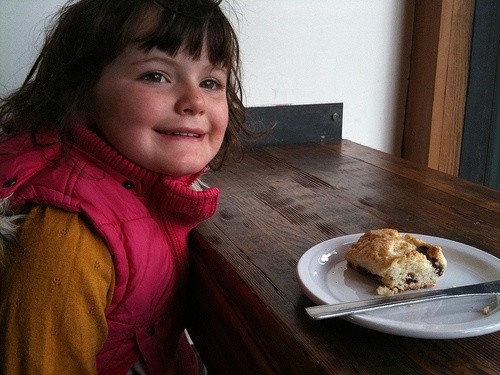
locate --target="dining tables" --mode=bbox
[186,137,500,375]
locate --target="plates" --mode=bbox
[296,232,500,339]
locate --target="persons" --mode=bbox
[0,0,278,375]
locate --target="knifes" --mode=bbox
[306,279,500,320]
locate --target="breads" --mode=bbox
[343,228,447,296]
